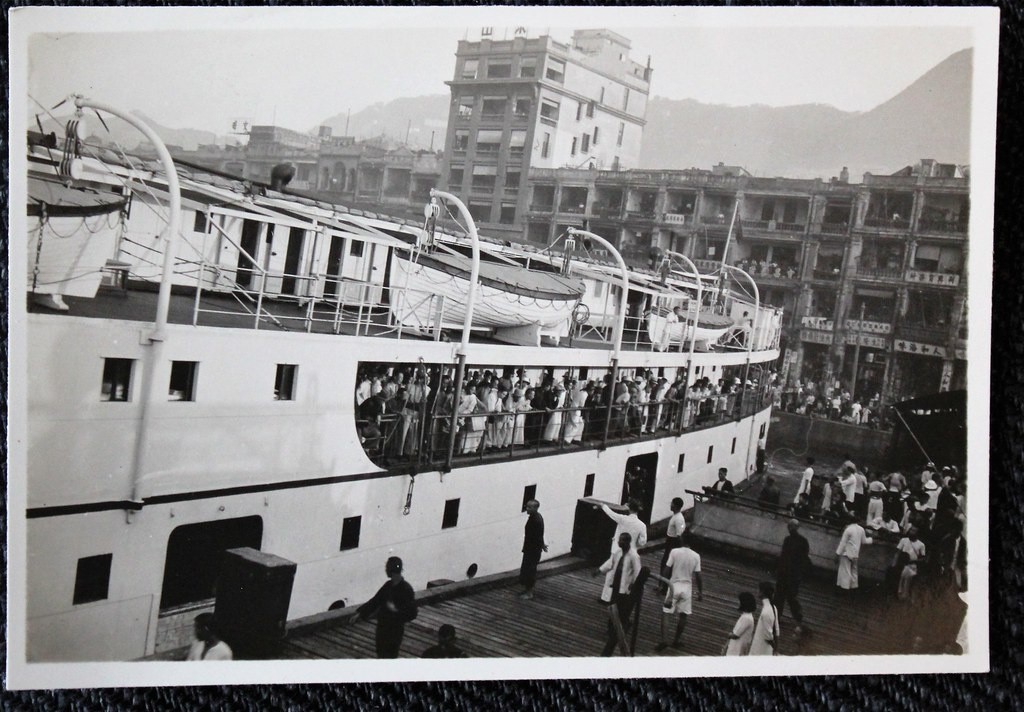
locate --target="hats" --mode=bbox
[386,557,403,570]
[924,479,938,490]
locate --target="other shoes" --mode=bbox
[656,642,667,653]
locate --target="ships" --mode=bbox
[27,133,786,663]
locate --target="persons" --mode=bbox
[705,467,735,500]
[516,499,549,600]
[832,515,875,596]
[746,380,753,389]
[767,368,880,425]
[771,520,813,635]
[748,582,780,655]
[355,366,535,456]
[186,613,233,659]
[794,451,966,552]
[599,498,647,605]
[657,306,681,351]
[525,374,597,446]
[742,257,779,268]
[721,593,757,656]
[654,497,686,595]
[593,367,684,440]
[727,311,750,351]
[349,557,418,659]
[683,377,741,430]
[787,493,810,518]
[759,475,779,507]
[891,526,925,599]
[654,530,702,650]
[592,532,642,635]
[421,623,470,658]
[937,519,966,572]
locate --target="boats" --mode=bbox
[645,305,733,352]
[391,244,586,343]
[26,170,127,307]
